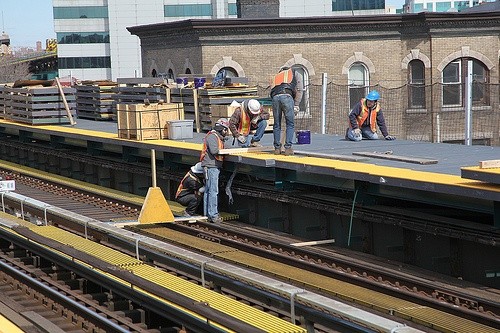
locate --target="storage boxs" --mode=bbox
[295,130,310,144]
[166,120,194,140]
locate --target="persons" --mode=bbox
[200,118,229,223]
[345,90,396,141]
[229,99,270,149]
[175,162,206,218]
[270,67,303,155]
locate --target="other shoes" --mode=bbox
[285,148,294,155]
[275,148,281,155]
[250,141,261,147]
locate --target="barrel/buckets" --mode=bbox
[194,77,206,89]
[296,129,310,145]
[176,77,189,86]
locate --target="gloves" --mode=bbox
[251,115,261,124]
[354,129,361,134]
[238,136,246,144]
[385,135,396,140]
[294,105,299,113]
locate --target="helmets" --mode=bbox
[248,99,260,114]
[191,162,203,173]
[215,118,229,128]
[366,90,380,100]
